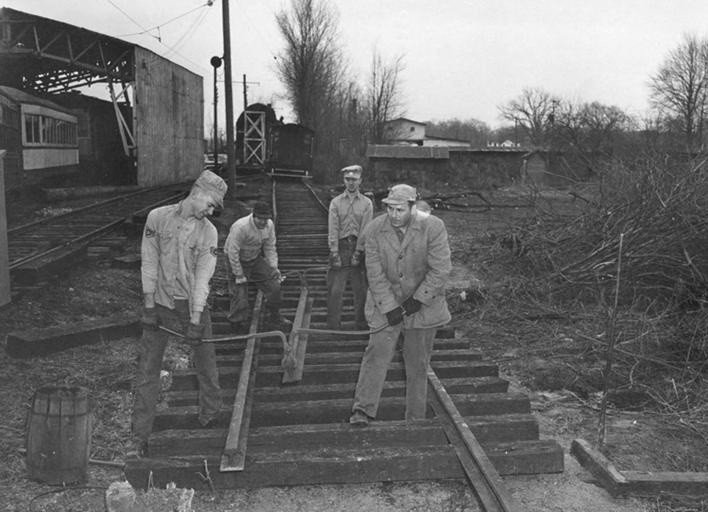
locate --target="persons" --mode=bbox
[323,165,374,332]
[123,169,230,463]
[222,200,295,335]
[346,183,455,427]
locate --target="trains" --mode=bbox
[266,124,315,180]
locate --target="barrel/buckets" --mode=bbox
[26,386,92,484]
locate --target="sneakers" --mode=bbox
[268,315,293,332]
[350,411,369,427]
[125,444,148,462]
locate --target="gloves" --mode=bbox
[235,274,248,286]
[185,322,206,346]
[141,307,162,331]
[271,267,286,285]
[350,251,363,267]
[386,307,404,326]
[402,295,422,316]
[330,251,342,271]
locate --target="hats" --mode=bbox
[342,165,362,179]
[381,184,416,205]
[195,170,227,209]
[253,201,272,219]
